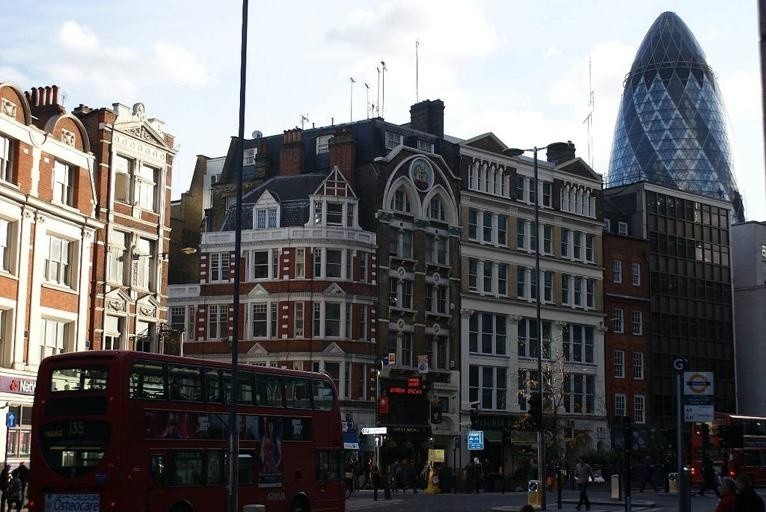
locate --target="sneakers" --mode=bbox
[585,503,590,511]
[575,506,581,511]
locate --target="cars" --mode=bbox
[344,463,354,501]
[628,451,670,476]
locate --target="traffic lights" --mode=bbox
[527,389,541,431]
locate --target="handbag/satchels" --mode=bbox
[345,472,353,480]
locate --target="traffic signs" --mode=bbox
[360,426,387,435]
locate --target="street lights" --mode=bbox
[372,352,389,465]
[499,139,577,511]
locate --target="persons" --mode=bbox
[161,422,182,439]
[574,456,595,511]
[345,456,539,497]
[0,462,30,512]
[238,415,255,440]
[261,418,282,474]
[639,455,766,512]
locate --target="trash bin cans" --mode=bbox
[528,480,542,510]
[666,473,680,493]
[611,474,620,502]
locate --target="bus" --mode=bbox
[26,349,346,512]
[688,412,766,490]
